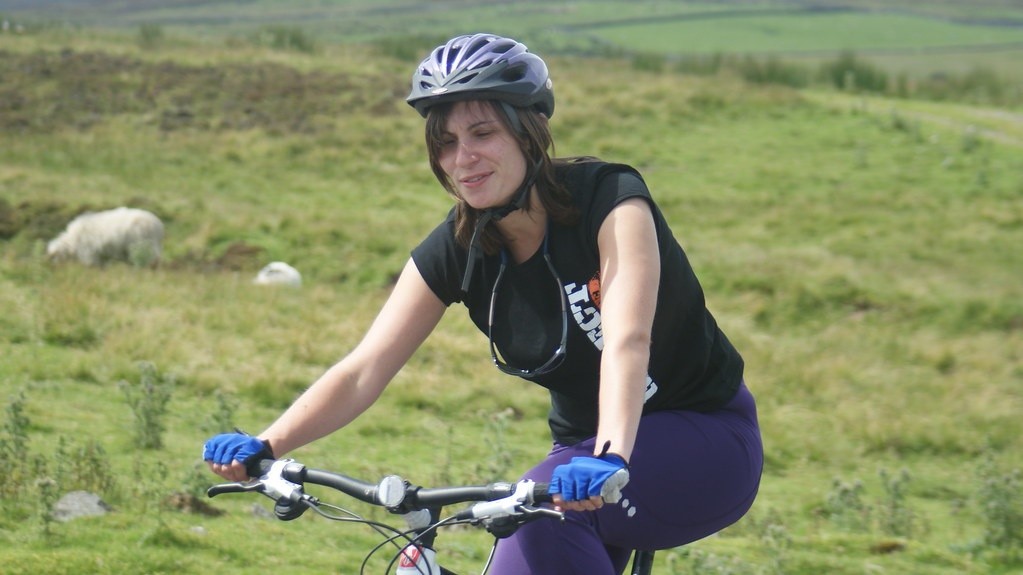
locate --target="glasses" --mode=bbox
[488,253,566,379]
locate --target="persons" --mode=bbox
[202,32,763,573]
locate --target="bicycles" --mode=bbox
[207,457,566,575]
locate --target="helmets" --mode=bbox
[406,32,554,121]
